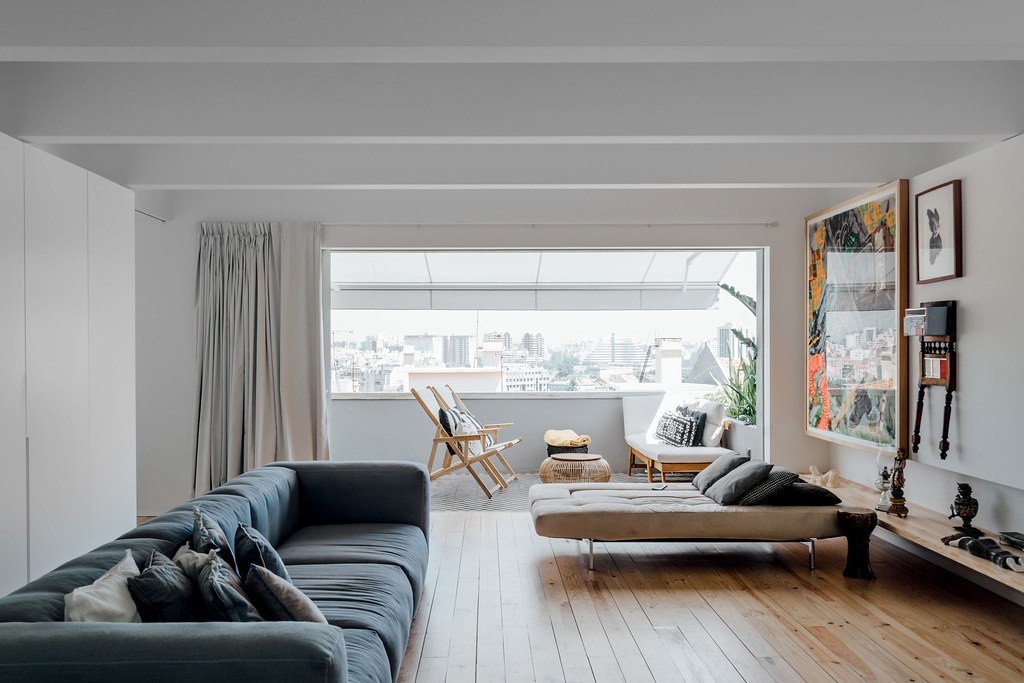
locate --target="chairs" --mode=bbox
[623,396,735,483]
[411,384,523,499]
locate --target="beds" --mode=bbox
[529,483,843,570]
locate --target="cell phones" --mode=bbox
[652,484,668,491]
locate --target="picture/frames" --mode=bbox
[804,179,909,456]
[915,180,963,284]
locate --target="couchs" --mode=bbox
[0,460,429,683]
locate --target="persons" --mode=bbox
[891,448,905,487]
[879,466,892,480]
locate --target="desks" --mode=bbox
[539,453,612,483]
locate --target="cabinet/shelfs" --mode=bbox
[1,132,137,600]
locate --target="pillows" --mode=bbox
[234,520,292,587]
[127,546,214,622]
[194,502,237,572]
[765,479,842,506]
[735,467,799,506]
[460,410,494,457]
[439,406,470,455]
[64,550,142,623]
[692,452,751,495]
[675,405,707,446]
[198,548,263,622]
[171,541,220,581]
[704,459,774,507]
[245,563,328,624]
[655,410,697,447]
